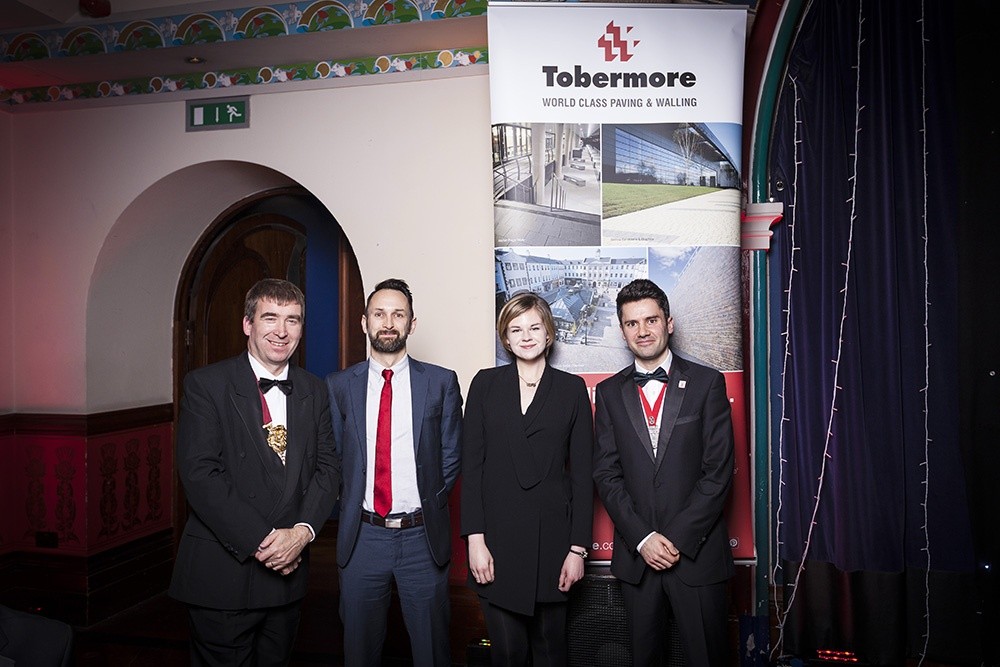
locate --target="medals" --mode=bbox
[637,380,671,447]
[254,376,289,458]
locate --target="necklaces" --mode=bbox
[518,374,541,386]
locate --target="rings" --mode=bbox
[271,561,276,567]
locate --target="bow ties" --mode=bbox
[257,377,293,396]
[633,367,669,388]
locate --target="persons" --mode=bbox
[164,279,339,667]
[460,288,596,665]
[326,278,465,666]
[593,278,738,667]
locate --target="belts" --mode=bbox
[361,510,424,529]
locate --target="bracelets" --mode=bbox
[568,549,588,558]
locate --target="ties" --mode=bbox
[373,369,394,519]
